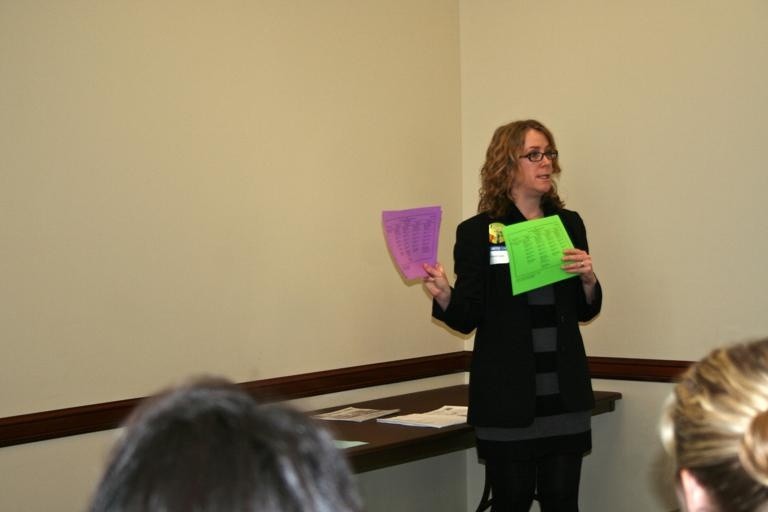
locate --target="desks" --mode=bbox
[303,385,622,512]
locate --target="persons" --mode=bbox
[91,375,366,512]
[658,338,767,511]
[422,119,603,512]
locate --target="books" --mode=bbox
[329,438,370,451]
[309,404,402,423]
[377,403,469,430]
[502,213,583,300]
[380,205,444,288]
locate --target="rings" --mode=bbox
[580,261,585,269]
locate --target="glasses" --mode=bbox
[517,148,559,161]
